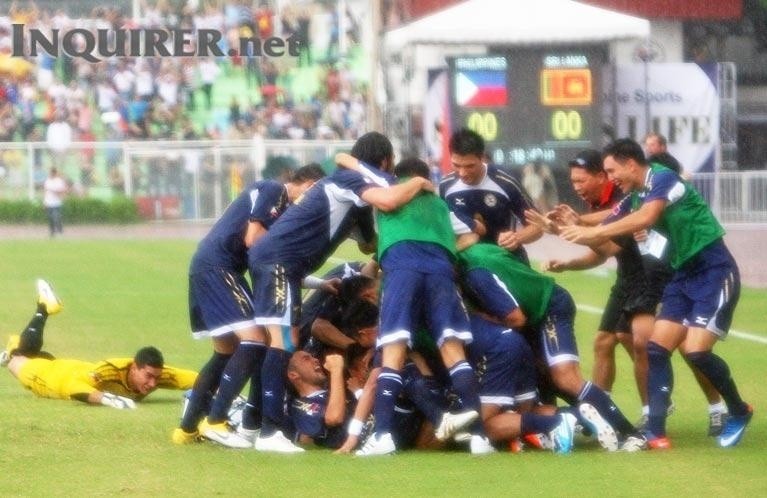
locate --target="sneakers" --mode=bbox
[0,333,22,368]
[719,403,754,449]
[618,400,677,452]
[36,279,64,316]
[354,430,397,458]
[434,403,578,456]
[707,411,730,438]
[172,422,306,455]
[576,402,619,453]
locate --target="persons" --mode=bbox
[644,134,679,173]
[0,277,200,412]
[553,138,753,447]
[44,169,66,238]
[555,152,727,440]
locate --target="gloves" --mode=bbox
[100,392,138,411]
